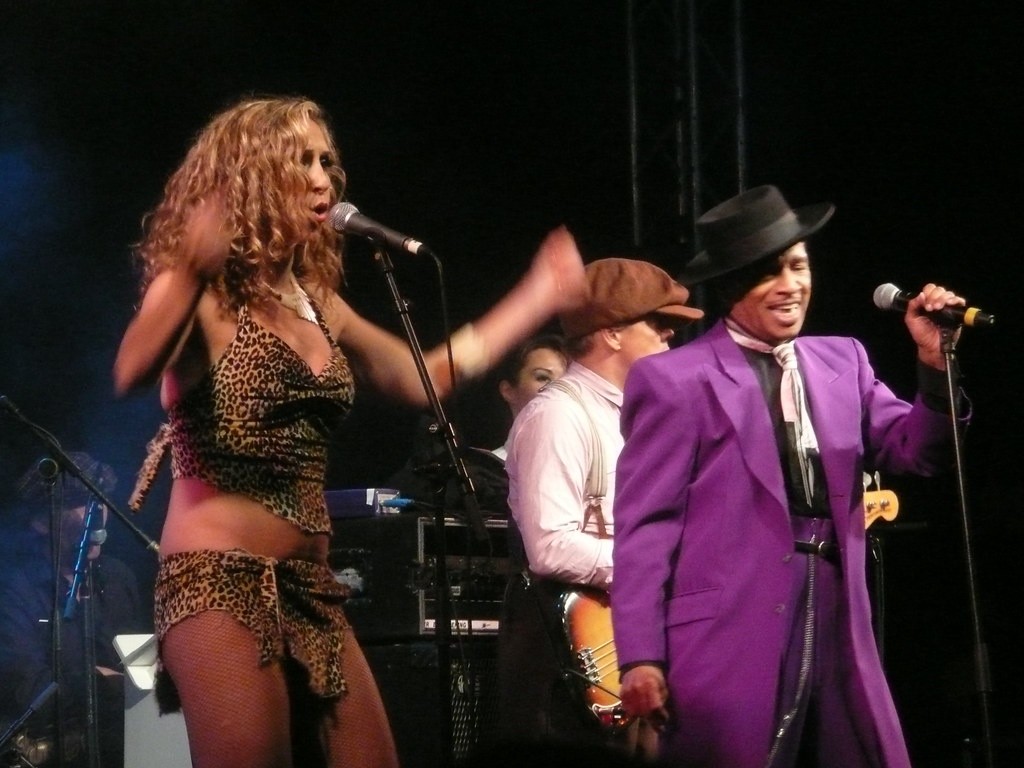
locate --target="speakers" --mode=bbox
[354,642,492,768]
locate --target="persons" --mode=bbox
[497,258,704,768]
[114,100,587,768]
[612,187,972,768]
[449,333,572,762]
[0,451,149,768]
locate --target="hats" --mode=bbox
[560,258,705,340]
[677,185,836,287]
[20,450,118,511]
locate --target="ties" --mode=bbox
[725,327,820,507]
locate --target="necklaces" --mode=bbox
[261,275,319,324]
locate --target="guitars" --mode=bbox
[556,469,899,734]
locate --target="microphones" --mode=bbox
[328,202,432,256]
[873,282,995,328]
[89,464,107,546]
[0,393,19,415]
